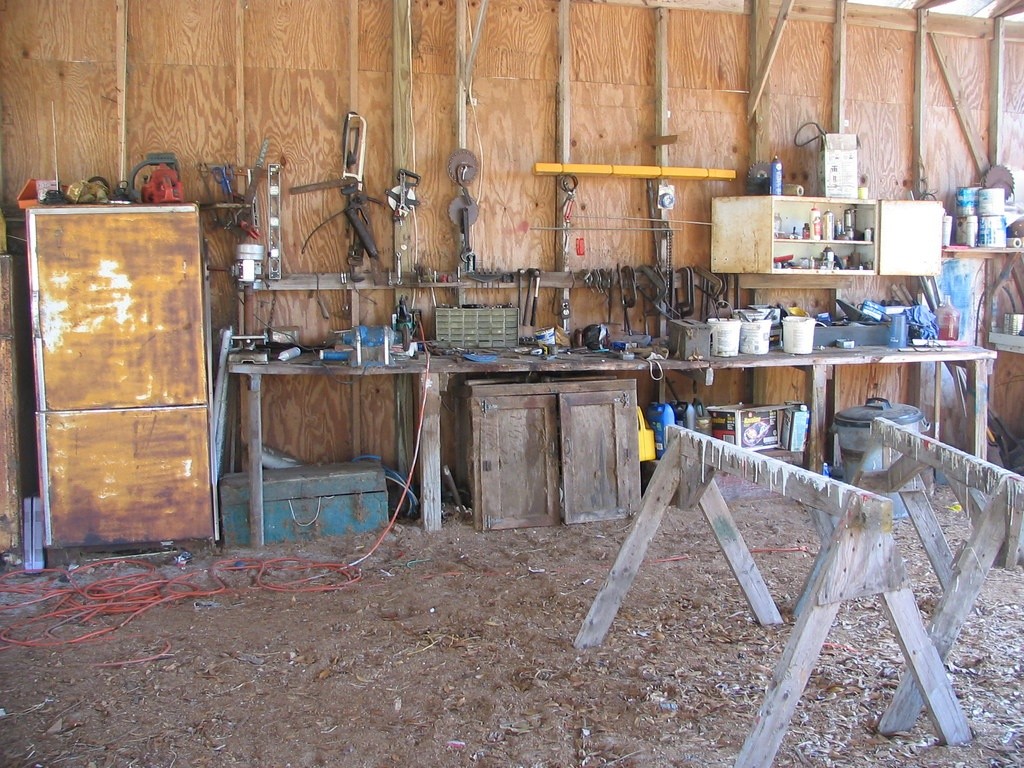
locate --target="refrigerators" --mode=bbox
[25,202,220,564]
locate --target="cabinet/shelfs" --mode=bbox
[25,201,219,550]
[0,253,26,558]
[452,378,642,532]
[711,196,943,276]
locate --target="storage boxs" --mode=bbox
[220,463,390,549]
[816,134,864,199]
[706,404,793,451]
[814,320,890,348]
[715,454,803,501]
[670,320,711,360]
[24,496,45,574]
[435,307,519,349]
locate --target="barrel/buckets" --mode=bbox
[781,316,815,354]
[740,317,772,354]
[690,397,713,438]
[707,317,741,357]
[667,399,696,432]
[636,406,655,461]
[957,187,1006,248]
[829,397,930,519]
[646,398,674,460]
[732,309,765,322]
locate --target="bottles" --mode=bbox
[822,244,835,270]
[932,295,960,340]
[774,212,782,239]
[769,155,782,195]
[809,208,821,241]
[823,209,834,240]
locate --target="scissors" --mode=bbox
[213,165,238,225]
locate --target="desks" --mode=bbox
[229,269,998,549]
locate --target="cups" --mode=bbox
[888,314,907,349]
